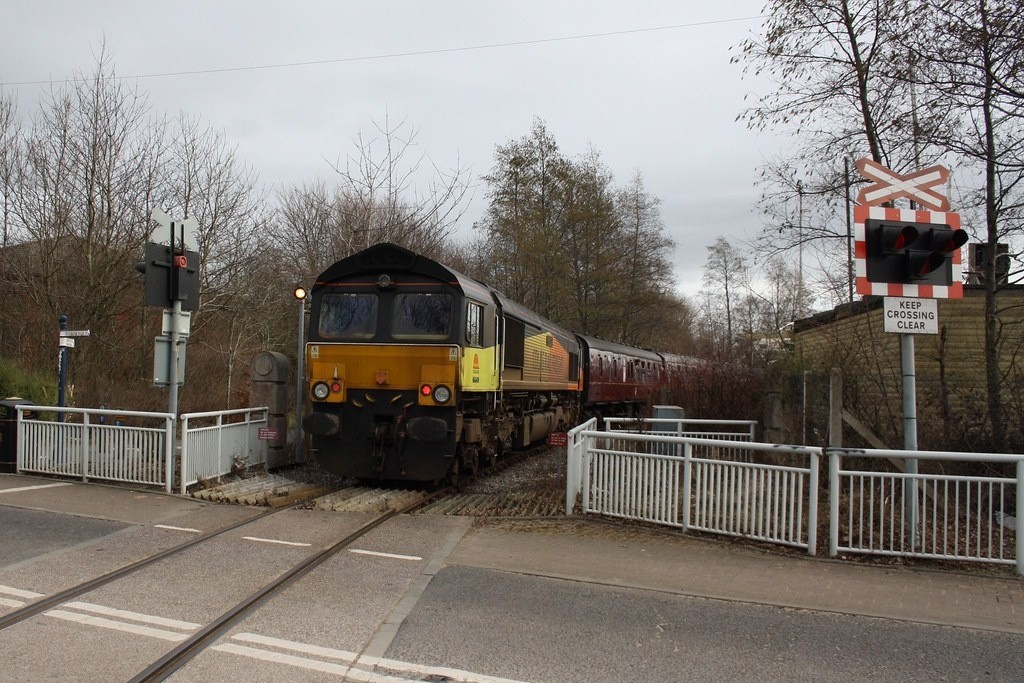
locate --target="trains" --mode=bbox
[302,241,762,494]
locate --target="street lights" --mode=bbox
[797,179,803,314]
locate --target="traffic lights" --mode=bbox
[865,220,968,287]
